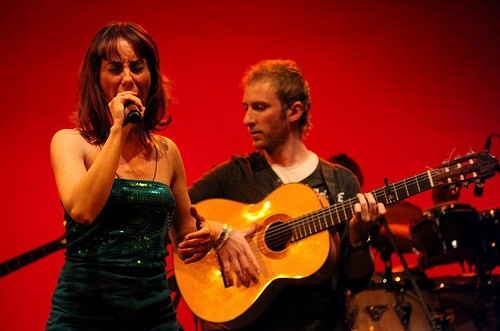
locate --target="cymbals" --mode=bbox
[371,200,425,255]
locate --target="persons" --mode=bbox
[45,22,214,331]
[187,60,387,331]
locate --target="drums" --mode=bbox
[344,272,445,331]
[411,200,480,258]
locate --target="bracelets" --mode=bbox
[352,235,372,249]
[214,223,232,250]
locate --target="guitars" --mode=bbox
[172,151,495,331]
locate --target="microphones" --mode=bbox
[474,136,491,196]
[124,103,142,124]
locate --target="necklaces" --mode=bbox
[99,132,157,183]
